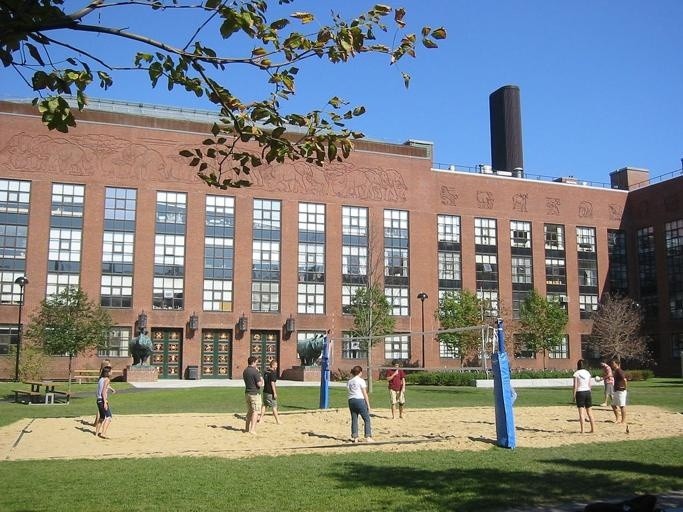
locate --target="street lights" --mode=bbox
[15,274,28,382]
[415,288,427,370]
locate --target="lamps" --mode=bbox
[238,311,247,333]
[137,309,148,334]
[189,310,198,332]
[285,313,295,335]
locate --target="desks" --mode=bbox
[20,379,64,406]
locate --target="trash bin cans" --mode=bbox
[186,366,199,380]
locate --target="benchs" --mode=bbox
[10,388,42,406]
[46,389,72,404]
[73,367,123,385]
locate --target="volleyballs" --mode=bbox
[595,375,601,381]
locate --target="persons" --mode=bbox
[242,356,263,435]
[257,359,284,426]
[510,385,517,406]
[610,358,628,424]
[95,366,113,440]
[570,358,596,435]
[92,361,116,428]
[385,358,406,420]
[346,365,375,444]
[596,361,615,406]
[102,358,116,395]
